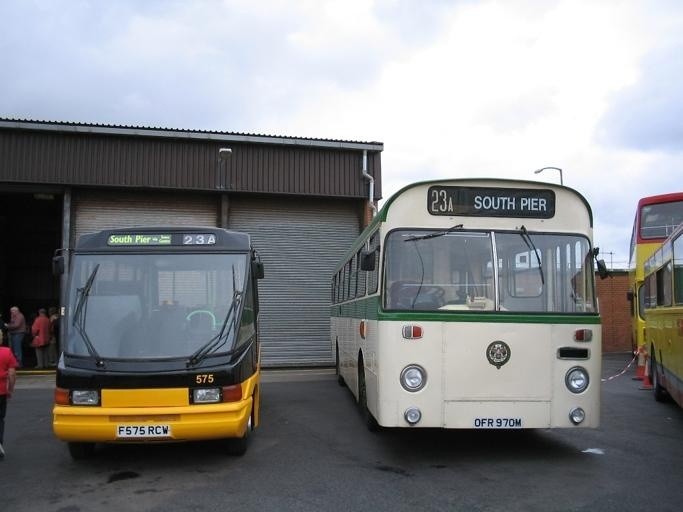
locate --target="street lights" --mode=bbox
[533,166,563,186]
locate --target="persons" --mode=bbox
[48,306,59,367]
[0,312,8,345]
[30,307,51,369]
[0,329,20,457]
[8,306,26,368]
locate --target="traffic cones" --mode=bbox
[637,352,653,390]
[631,346,645,381]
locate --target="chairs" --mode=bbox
[391,281,424,309]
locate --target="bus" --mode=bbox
[330,176,608,433]
[624,193,683,357]
[51,226,266,454]
[641,221,683,410]
